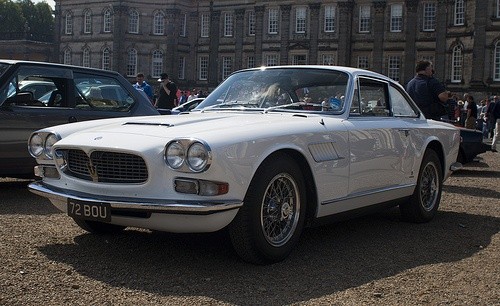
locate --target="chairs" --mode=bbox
[11,92,32,106]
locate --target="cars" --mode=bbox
[29,65,462,265]
[0,58,160,182]
[169,98,248,114]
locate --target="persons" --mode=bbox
[406,60,448,122]
[89,71,500,152]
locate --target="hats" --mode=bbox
[85,87,104,100]
